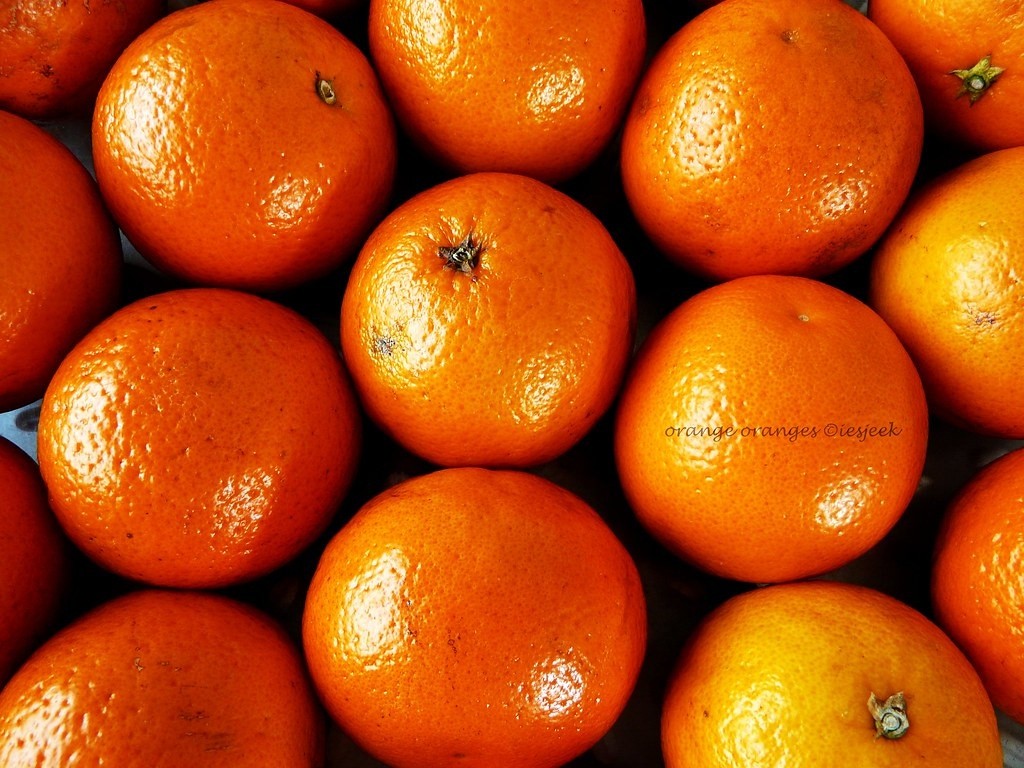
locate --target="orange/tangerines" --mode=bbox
[0,0,1024,768]
